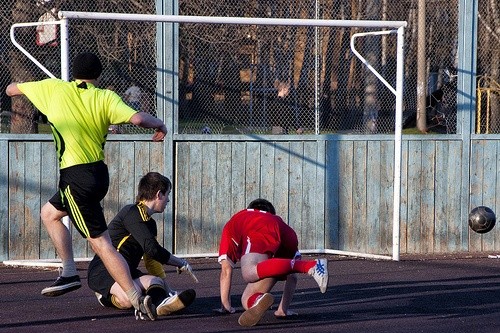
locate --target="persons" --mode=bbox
[124,80,143,111]
[5,52,168,320]
[213,199,329,326]
[87,172,198,316]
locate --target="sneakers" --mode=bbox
[237,292,274,327]
[155,288,196,316]
[94,291,108,307]
[40,274,82,297]
[308,256,329,294]
[136,294,156,322]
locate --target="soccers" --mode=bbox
[468,206,496,234]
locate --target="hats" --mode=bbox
[72,52,103,80]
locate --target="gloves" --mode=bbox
[176,260,198,284]
